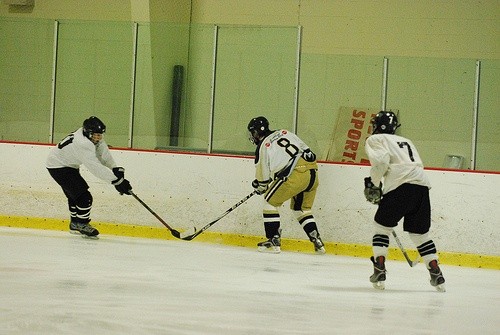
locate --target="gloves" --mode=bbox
[112,166,125,180]
[111,177,132,196]
[251,177,273,195]
[363,176,382,204]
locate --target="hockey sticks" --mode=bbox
[171,190,257,241]
[377,200,419,267]
[129,189,196,239]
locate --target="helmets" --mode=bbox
[82,116,106,145]
[370,110,398,135]
[247,116,269,144]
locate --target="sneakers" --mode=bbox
[429,267,447,292]
[368,255,387,290]
[67,205,100,240]
[257,229,282,253]
[309,229,326,254]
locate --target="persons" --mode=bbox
[247,116,324,251]
[46,116,132,236]
[364,111,445,286]
